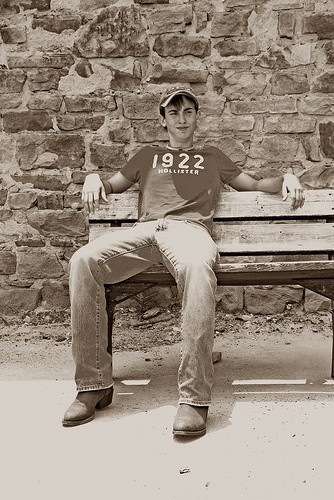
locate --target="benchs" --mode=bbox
[88,189,334,379]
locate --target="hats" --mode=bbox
[157,86,199,114]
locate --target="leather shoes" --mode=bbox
[62,384,114,427]
[171,402,208,436]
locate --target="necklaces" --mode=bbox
[167,144,195,152]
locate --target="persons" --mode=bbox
[61,86,306,438]
